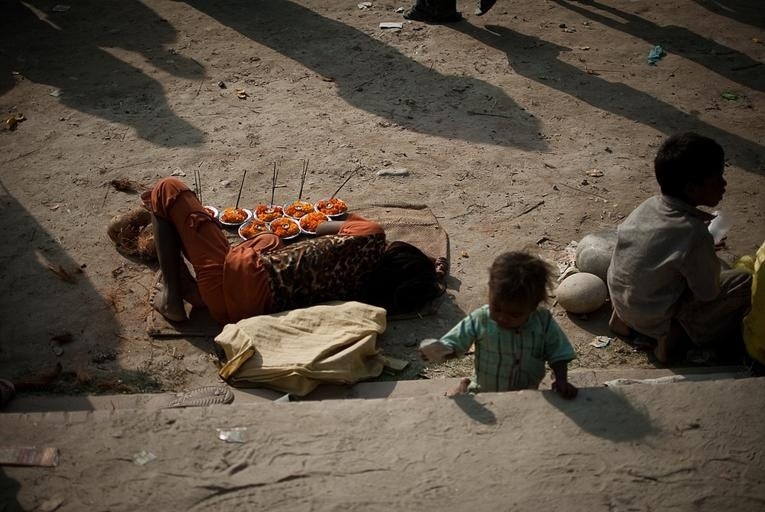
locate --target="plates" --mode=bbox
[203,199,350,240]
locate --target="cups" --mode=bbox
[708,210,733,245]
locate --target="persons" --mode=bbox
[147,176,448,326]
[418,251,580,399]
[606,132,753,367]
